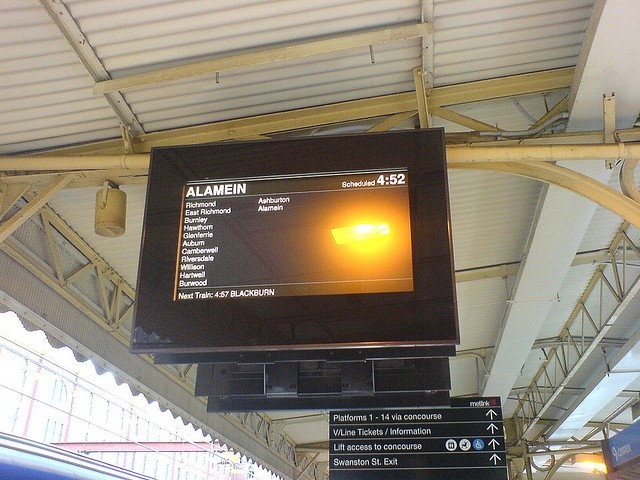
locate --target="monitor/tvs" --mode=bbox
[127,127,463,359]
[194,362,453,412]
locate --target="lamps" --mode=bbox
[94,182,126,238]
[574,452,608,473]
